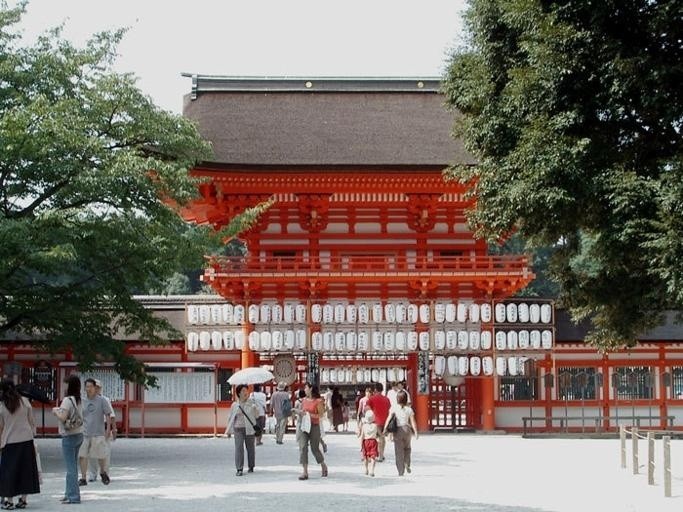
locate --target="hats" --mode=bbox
[95,379,101,387]
[365,410,375,423]
[277,382,287,391]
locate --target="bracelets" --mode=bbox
[112,429,117,432]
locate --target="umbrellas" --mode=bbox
[226,367,275,386]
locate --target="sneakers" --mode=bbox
[101,472,109,484]
[78,479,87,486]
[1,501,14,510]
[16,499,26,508]
[90,472,96,481]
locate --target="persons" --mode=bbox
[0,378,41,510]
[224,382,419,480]
[88,380,117,483]
[77,378,113,485]
[51,374,84,504]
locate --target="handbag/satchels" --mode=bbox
[387,416,397,432]
[254,424,262,436]
[64,408,83,430]
[282,399,291,416]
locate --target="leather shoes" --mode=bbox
[237,469,243,476]
[248,467,253,473]
